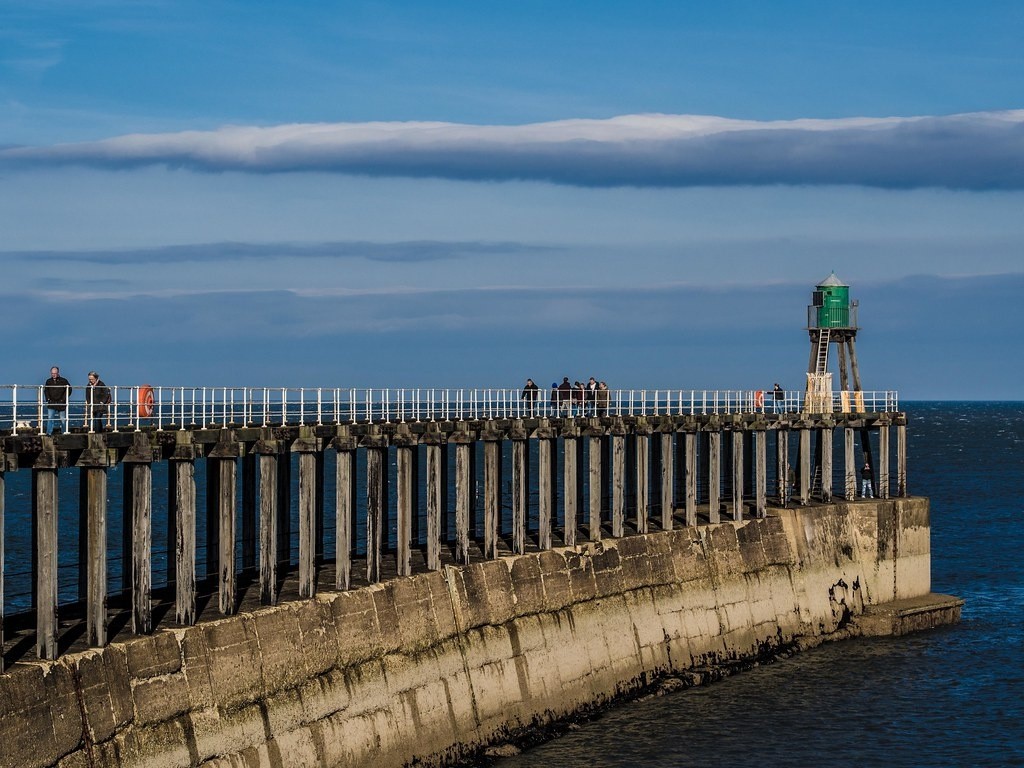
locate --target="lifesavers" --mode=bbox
[755,389,765,408]
[136,384,155,419]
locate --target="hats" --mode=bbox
[552,383,557,388]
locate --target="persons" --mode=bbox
[765,383,785,414]
[521,378,539,418]
[787,461,797,508]
[43,366,73,435]
[86,371,112,432]
[550,376,612,417]
[860,463,876,499]
